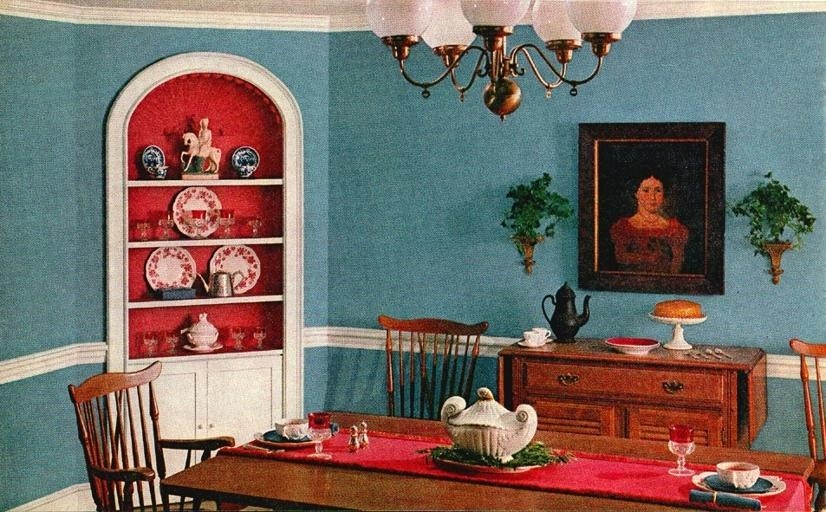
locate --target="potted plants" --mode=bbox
[501,165,576,279]
[729,170,819,287]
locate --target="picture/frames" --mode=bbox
[572,121,728,297]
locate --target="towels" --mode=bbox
[685,485,764,511]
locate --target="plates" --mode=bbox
[253,422,339,448]
[691,471,787,499]
[140,145,166,173]
[546,339,553,343]
[517,341,546,348]
[229,146,260,174]
[434,455,547,475]
[172,186,222,240]
[209,246,261,294]
[144,247,196,292]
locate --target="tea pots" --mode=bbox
[193,269,244,297]
[541,281,592,344]
[180,313,219,349]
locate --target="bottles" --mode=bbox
[346,425,360,452]
[358,419,370,448]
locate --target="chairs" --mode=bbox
[69,360,237,510]
[788,336,826,463]
[374,314,492,420]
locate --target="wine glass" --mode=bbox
[230,326,246,351]
[218,208,236,239]
[158,218,173,241]
[305,412,333,461]
[141,332,157,357]
[190,209,207,240]
[247,215,263,238]
[667,424,696,477]
[253,326,267,350]
[164,333,179,355]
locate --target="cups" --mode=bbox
[147,165,169,179]
[531,327,551,341]
[716,461,761,489]
[235,165,257,179]
[274,418,308,440]
[523,331,545,345]
[136,222,152,241]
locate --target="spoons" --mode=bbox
[241,444,286,455]
[683,348,733,359]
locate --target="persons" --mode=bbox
[199,119,212,155]
[609,170,689,273]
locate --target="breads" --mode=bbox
[655,300,702,318]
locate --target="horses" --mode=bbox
[178,131,224,175]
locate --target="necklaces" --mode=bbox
[638,210,659,223]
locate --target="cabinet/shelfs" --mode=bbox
[497,337,771,450]
[101,48,307,511]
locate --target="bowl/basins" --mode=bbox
[183,342,223,353]
[605,337,662,355]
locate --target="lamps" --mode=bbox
[364,1,639,121]
